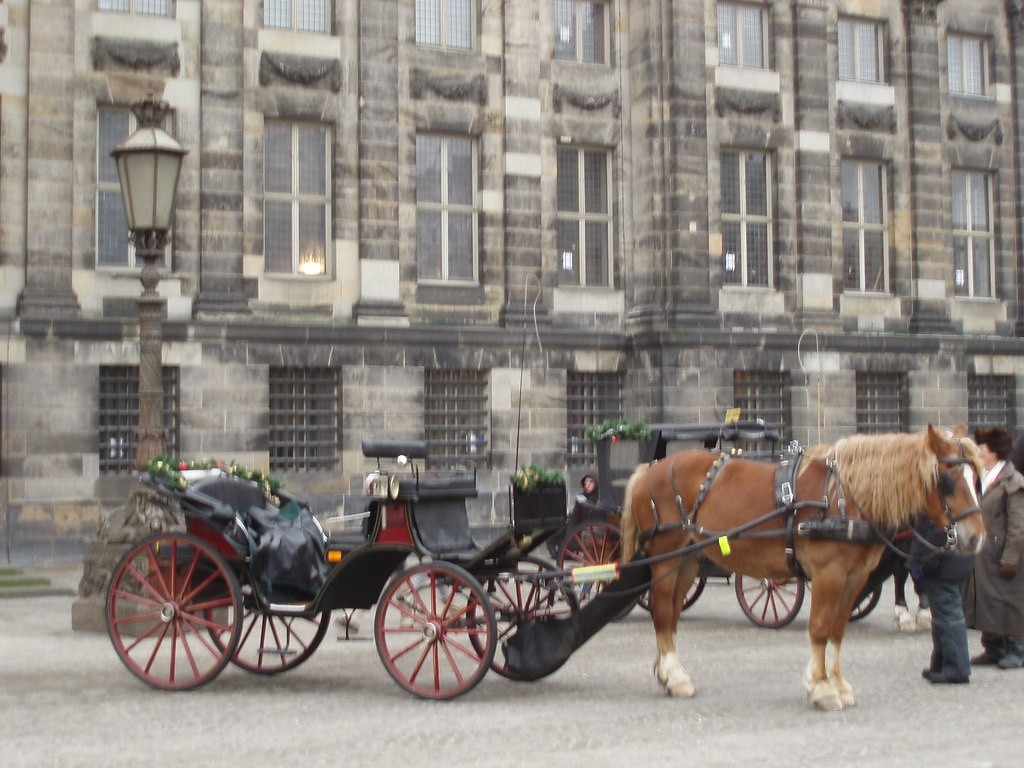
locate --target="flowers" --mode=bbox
[146,456,281,506]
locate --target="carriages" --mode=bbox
[557,413,1023,635]
[102,420,988,717]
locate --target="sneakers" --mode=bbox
[922,667,969,684]
[972,651,1024,669]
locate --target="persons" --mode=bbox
[911,427,1024,683]
[567,472,599,602]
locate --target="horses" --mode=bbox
[617,421,988,715]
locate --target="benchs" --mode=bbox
[403,478,487,551]
[182,476,272,532]
[326,438,428,548]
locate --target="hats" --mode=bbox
[974,427,1013,460]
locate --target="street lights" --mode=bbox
[72,93,231,638]
